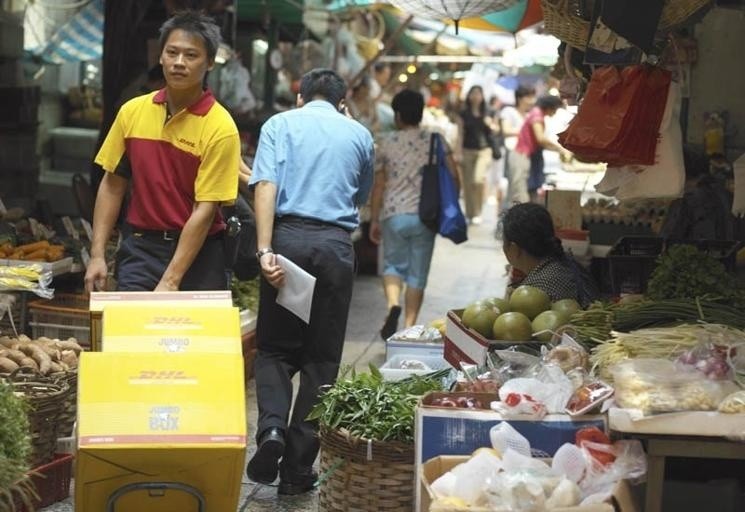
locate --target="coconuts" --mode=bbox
[461,284,581,342]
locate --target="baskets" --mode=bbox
[604,234,744,298]
[318,419,417,511]
[0,364,77,439]
[0,378,71,471]
[28,289,92,348]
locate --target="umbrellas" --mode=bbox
[443,0,545,49]
[388,0,524,35]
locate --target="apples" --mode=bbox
[523,394,532,401]
[506,392,521,406]
[532,404,541,412]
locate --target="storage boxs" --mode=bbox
[545,188,595,269]
[72,288,249,512]
[387,308,608,509]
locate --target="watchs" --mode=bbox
[255,248,273,264]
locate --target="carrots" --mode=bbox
[0,240,65,261]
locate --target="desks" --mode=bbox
[604,391,745,511]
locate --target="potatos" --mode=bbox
[0,334,83,375]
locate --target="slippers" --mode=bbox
[381,305,402,341]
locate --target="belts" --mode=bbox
[124,224,181,242]
[276,214,331,226]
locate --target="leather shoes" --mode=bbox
[246,426,287,486]
[277,469,319,495]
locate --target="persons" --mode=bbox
[114,65,167,120]
[369,89,461,340]
[83,8,241,294]
[457,84,573,226]
[247,67,376,495]
[222,156,260,281]
[495,200,603,308]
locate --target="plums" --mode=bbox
[430,396,482,408]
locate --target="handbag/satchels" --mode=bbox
[435,133,468,245]
[487,133,503,160]
[418,132,440,232]
